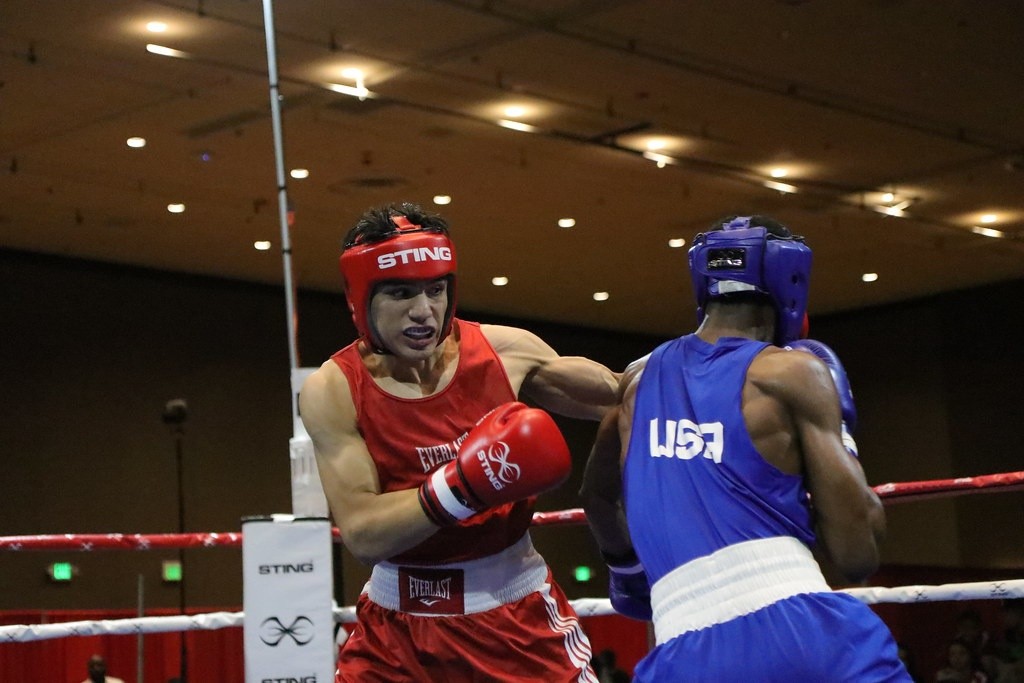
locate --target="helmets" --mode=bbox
[340,215,459,355]
[688,215,813,348]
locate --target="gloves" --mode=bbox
[607,561,653,626]
[424,402,570,523]
[786,339,858,455]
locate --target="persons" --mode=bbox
[81,654,123,683]
[588,648,632,683]
[897,608,1024,683]
[573,214,919,683]
[297,199,627,683]
